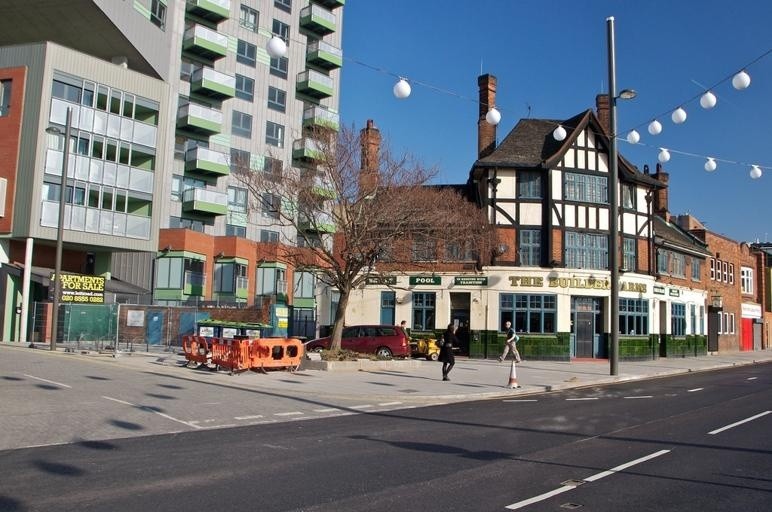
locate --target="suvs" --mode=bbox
[302,325,410,361]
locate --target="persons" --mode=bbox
[401,320,409,360]
[438,324,460,381]
[496,320,522,363]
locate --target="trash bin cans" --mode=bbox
[195,319,273,348]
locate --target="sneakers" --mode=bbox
[443,375,451,381]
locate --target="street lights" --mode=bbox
[45,106,74,351]
[610,88,636,375]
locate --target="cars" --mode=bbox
[406,329,442,361]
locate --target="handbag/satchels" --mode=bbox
[514,335,520,343]
[435,335,444,348]
